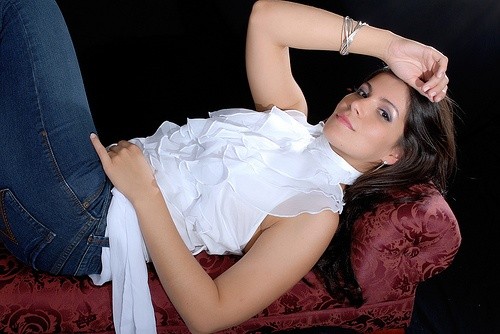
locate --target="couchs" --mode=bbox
[0,176,462,334]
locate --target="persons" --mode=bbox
[1,1,456,334]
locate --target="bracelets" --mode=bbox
[340,16,369,55]
